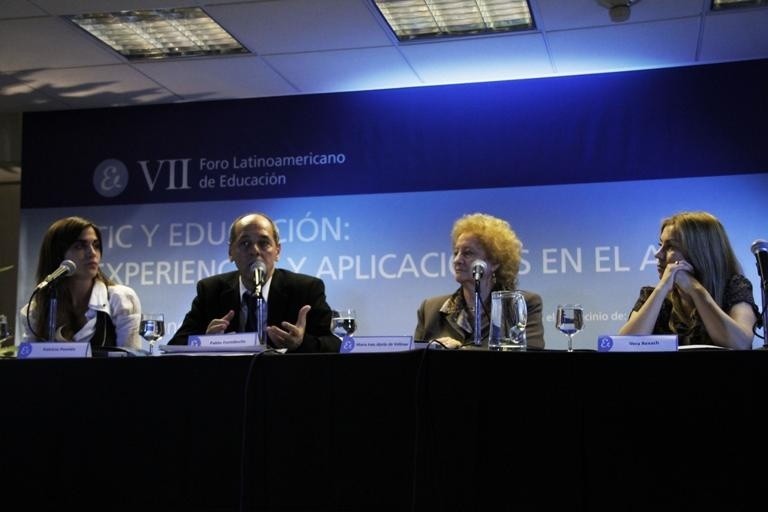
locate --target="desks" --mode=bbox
[1,345,767,511]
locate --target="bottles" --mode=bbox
[0,315,15,360]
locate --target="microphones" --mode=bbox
[471,259,488,281]
[750,238,768,279]
[250,260,266,297]
[36,259,77,292]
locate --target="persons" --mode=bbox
[413,213,546,350]
[20,216,143,352]
[619,211,764,351]
[167,212,342,354]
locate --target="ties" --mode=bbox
[243,290,263,332]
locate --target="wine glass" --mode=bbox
[555,302,582,356]
[137,313,167,359]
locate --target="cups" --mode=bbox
[330,309,357,341]
[487,292,529,351]
